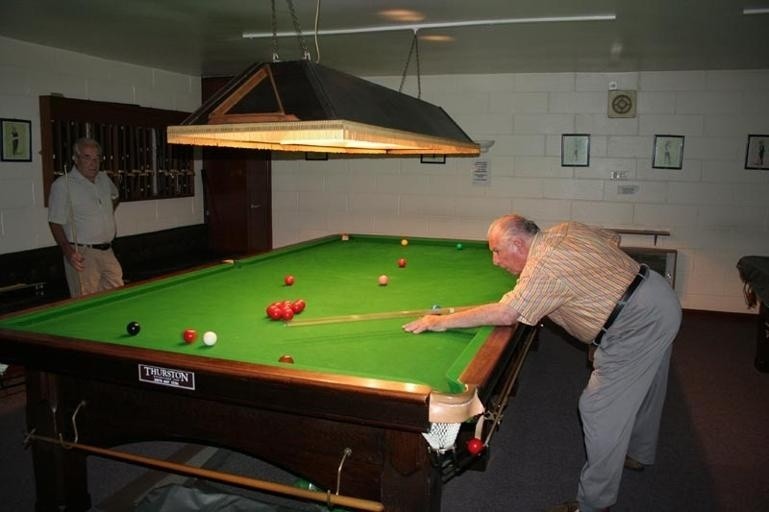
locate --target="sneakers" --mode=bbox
[563,501,609,512]
[624,456,644,471]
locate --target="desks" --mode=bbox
[736,256,769,373]
[0,234,539,512]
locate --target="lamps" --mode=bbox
[166,0,483,156]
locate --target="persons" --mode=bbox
[402,214,684,512]
[48,139,124,301]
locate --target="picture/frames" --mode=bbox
[420,155,446,164]
[745,134,769,170]
[561,133,590,167]
[652,134,685,169]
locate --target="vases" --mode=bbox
[305,153,327,160]
[0,118,32,163]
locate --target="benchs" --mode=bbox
[0,224,232,317]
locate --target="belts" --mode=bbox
[69,242,112,250]
[590,263,648,347]
